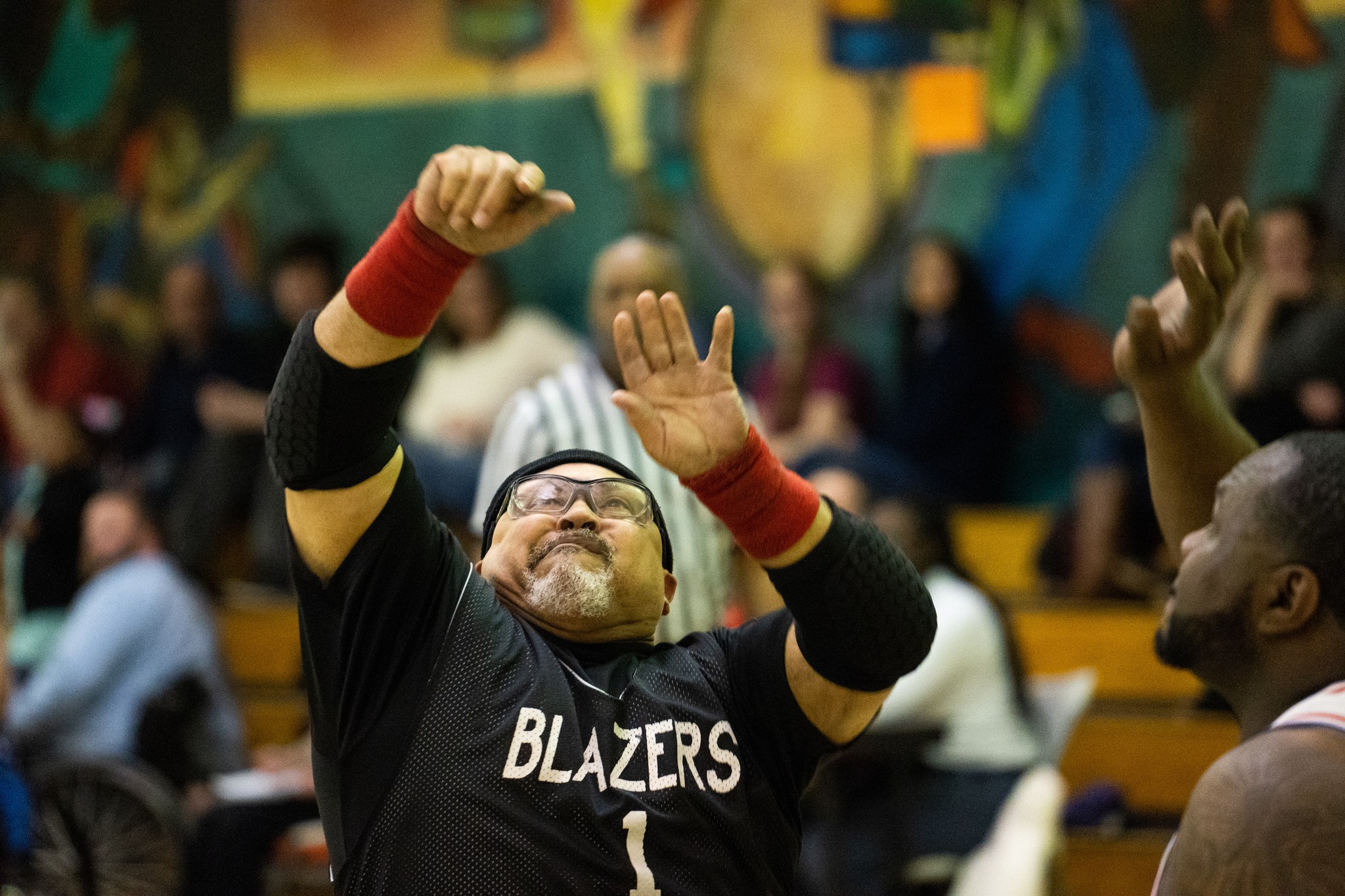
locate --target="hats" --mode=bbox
[481,448,674,574]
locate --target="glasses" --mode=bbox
[495,473,654,527]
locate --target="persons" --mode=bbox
[0,110,1345,896]
[1111,191,1345,896]
[266,143,937,896]
